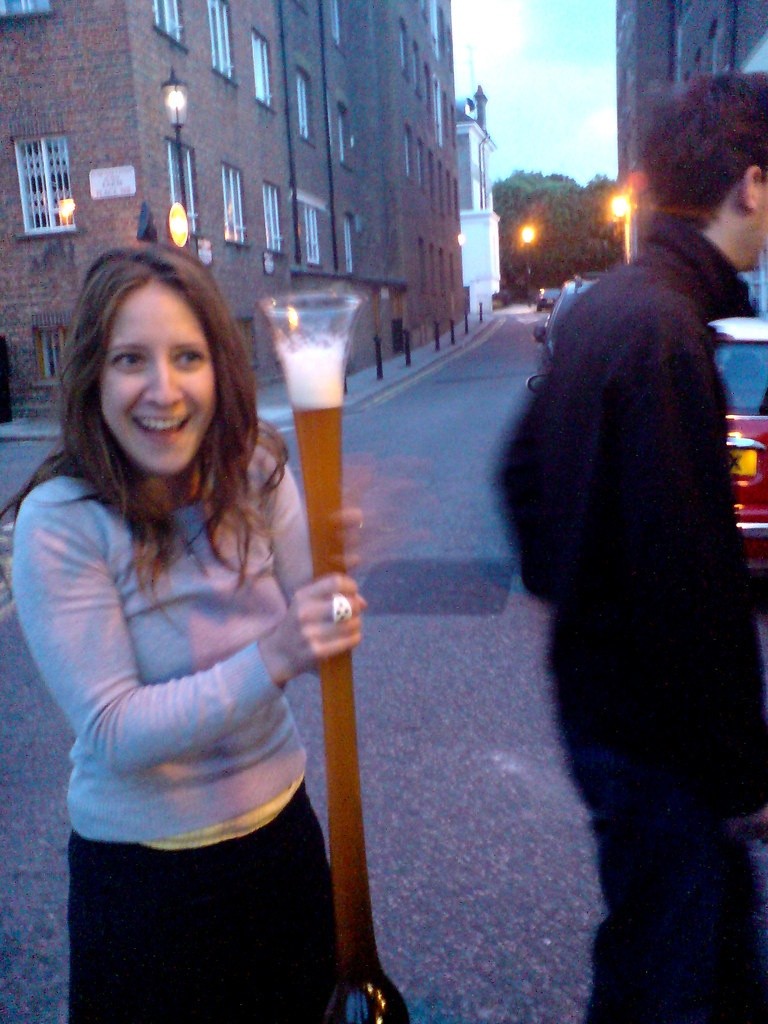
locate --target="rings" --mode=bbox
[330,592,353,623]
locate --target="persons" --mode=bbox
[9,241,371,1024]
[495,67,768,1024]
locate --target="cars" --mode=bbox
[537,287,562,311]
[526,272,768,578]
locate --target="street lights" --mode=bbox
[613,196,631,268]
[522,226,534,308]
[161,65,196,255]
